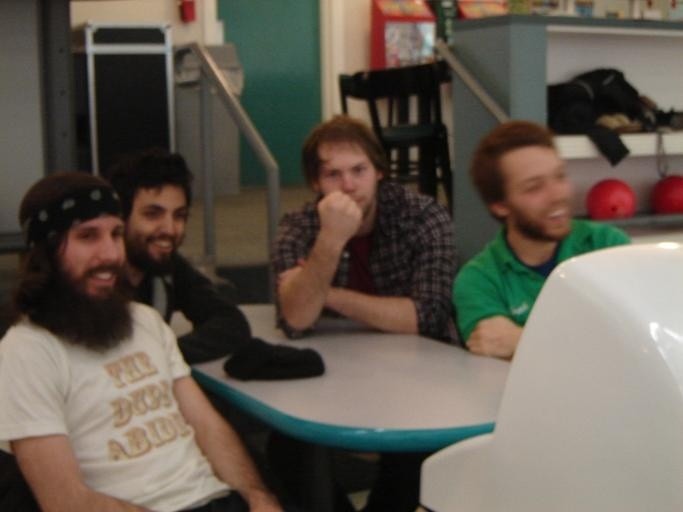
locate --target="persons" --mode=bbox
[0,149,254,512]
[0,167,288,512]
[449,117,634,360]
[264,114,458,512]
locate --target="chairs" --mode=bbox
[339,60,452,209]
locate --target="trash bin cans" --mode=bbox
[172,42,245,195]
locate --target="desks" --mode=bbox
[188,304,511,511]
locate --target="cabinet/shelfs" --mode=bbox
[453,14,682,264]
